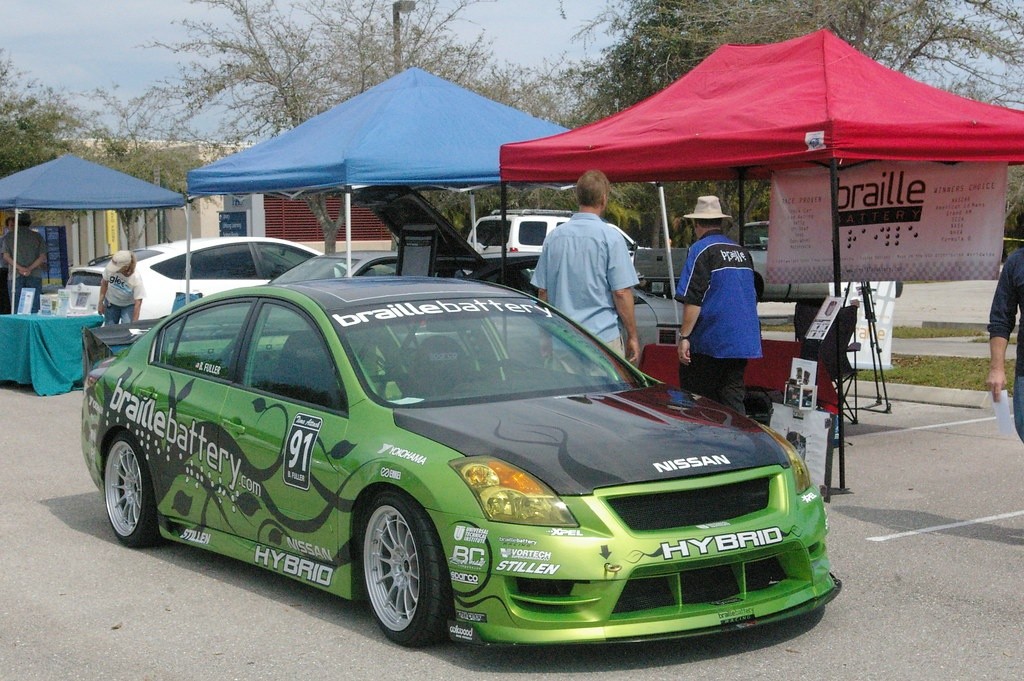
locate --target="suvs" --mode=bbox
[466,210,651,261]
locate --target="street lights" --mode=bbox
[392,0,416,75]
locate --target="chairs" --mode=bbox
[792,298,862,425]
[276,329,334,391]
[414,337,469,394]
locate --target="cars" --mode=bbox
[267,185,688,368]
[63,236,349,320]
[78,276,844,650]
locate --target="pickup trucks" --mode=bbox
[633,220,772,302]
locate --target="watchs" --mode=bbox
[678,332,689,340]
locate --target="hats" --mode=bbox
[106,250,131,272]
[683,195,732,219]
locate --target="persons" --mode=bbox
[98,250,143,325]
[530,170,639,377]
[674,196,763,414]
[0,213,47,314]
[986,248,1024,440]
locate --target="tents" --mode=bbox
[0,153,191,315]
[187,67,571,305]
[498,29,1024,347]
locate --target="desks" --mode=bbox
[0,315,104,397]
[639,342,837,417]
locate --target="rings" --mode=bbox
[680,357,682,358]
